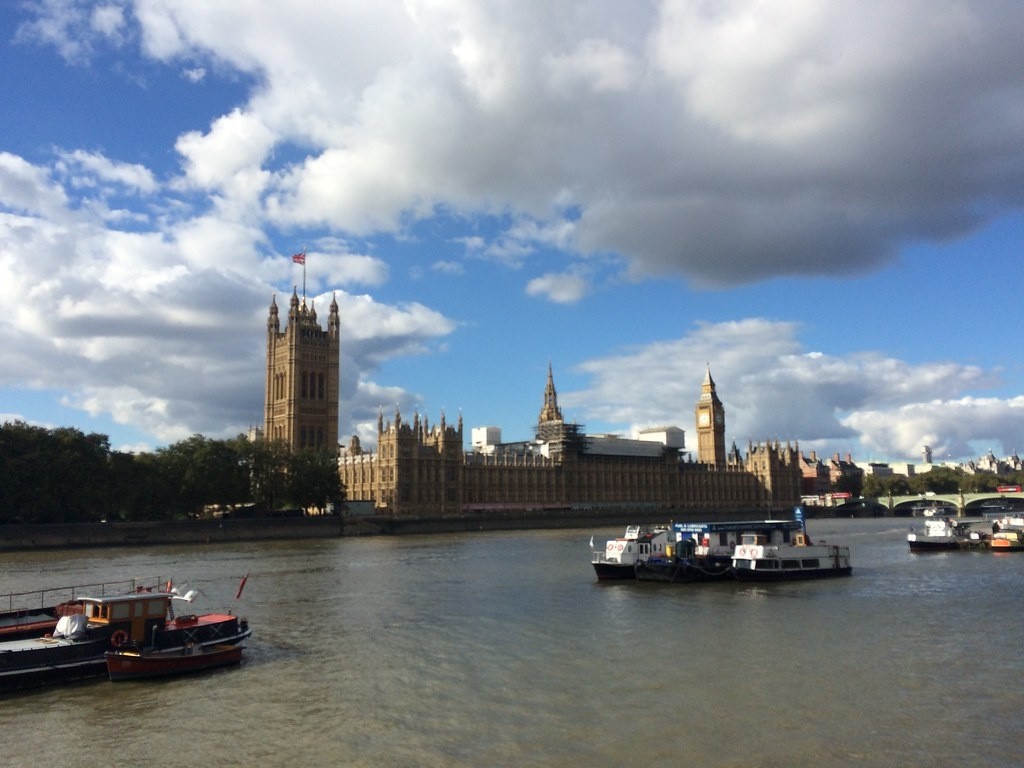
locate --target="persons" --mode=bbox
[992,520,1000,535]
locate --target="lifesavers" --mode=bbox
[111,629,128,648]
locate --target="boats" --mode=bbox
[989,512,1024,550]
[0,567,252,694]
[906,507,973,549]
[105,642,245,681]
[588,519,854,583]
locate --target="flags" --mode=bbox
[292,253,305,265]
